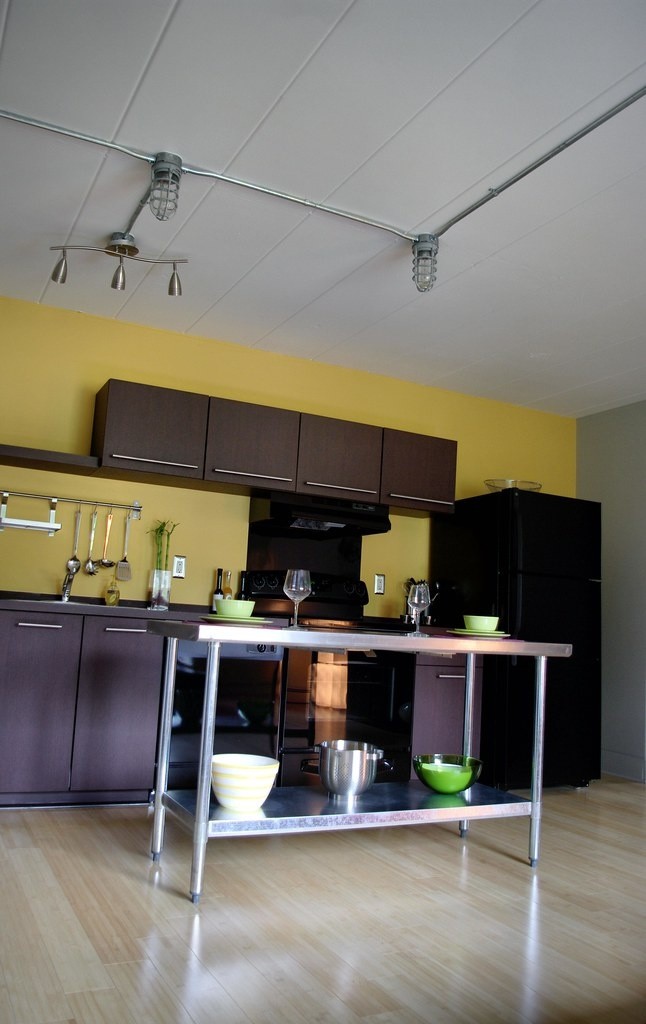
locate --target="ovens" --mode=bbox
[285,620,418,788]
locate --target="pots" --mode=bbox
[279,739,384,796]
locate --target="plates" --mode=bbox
[199,614,273,626]
[446,628,511,639]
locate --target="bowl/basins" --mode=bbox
[412,753,483,795]
[462,615,500,630]
[214,598,255,618]
[209,753,280,812]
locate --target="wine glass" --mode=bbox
[407,584,431,638]
[282,568,312,633]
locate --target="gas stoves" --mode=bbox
[249,485,391,542]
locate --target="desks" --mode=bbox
[145,620,572,902]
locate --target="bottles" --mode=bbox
[211,568,224,613]
[224,571,232,601]
[236,570,250,602]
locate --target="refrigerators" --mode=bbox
[429,487,601,793]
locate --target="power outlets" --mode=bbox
[173,554,186,579]
[374,573,385,595]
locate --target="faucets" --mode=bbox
[61,568,77,602]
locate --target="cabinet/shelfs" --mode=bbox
[93,379,457,515]
[0,609,166,806]
[410,625,483,779]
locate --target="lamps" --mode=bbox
[50,246,189,297]
[411,232,440,294]
[150,153,182,222]
